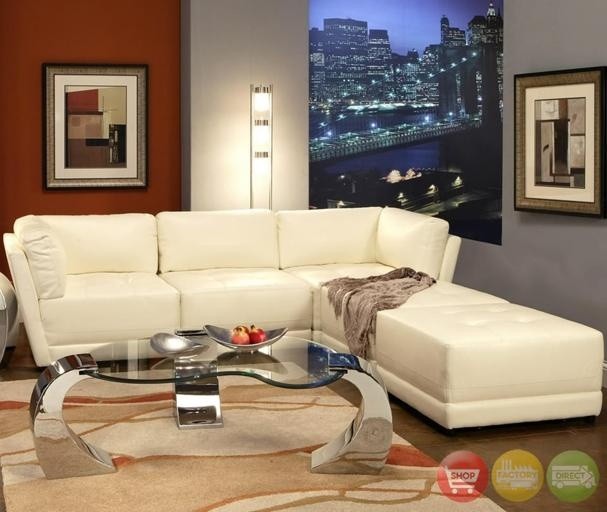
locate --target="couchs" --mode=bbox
[3,206,462,368]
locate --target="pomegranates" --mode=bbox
[232,324,266,344]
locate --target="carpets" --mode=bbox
[0,362,507,512]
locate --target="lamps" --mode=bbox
[250,84,274,210]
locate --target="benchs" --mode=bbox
[321,282,604,430]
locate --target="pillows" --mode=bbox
[13,206,450,300]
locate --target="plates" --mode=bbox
[203,324,288,351]
[150,331,209,362]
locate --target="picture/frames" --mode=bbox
[514,66,607,218]
[41,63,148,191]
